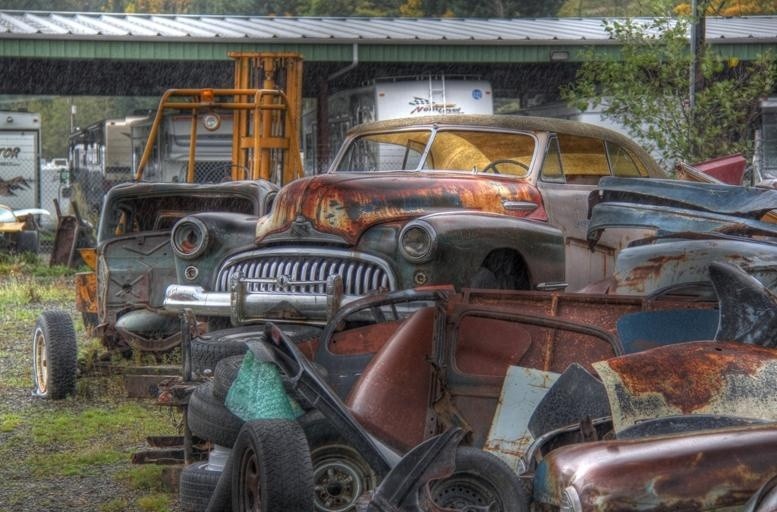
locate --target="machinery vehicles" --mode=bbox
[31,52,309,402]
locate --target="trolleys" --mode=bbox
[49,196,94,269]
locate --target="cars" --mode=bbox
[0,205,56,257]
[162,115,671,323]
